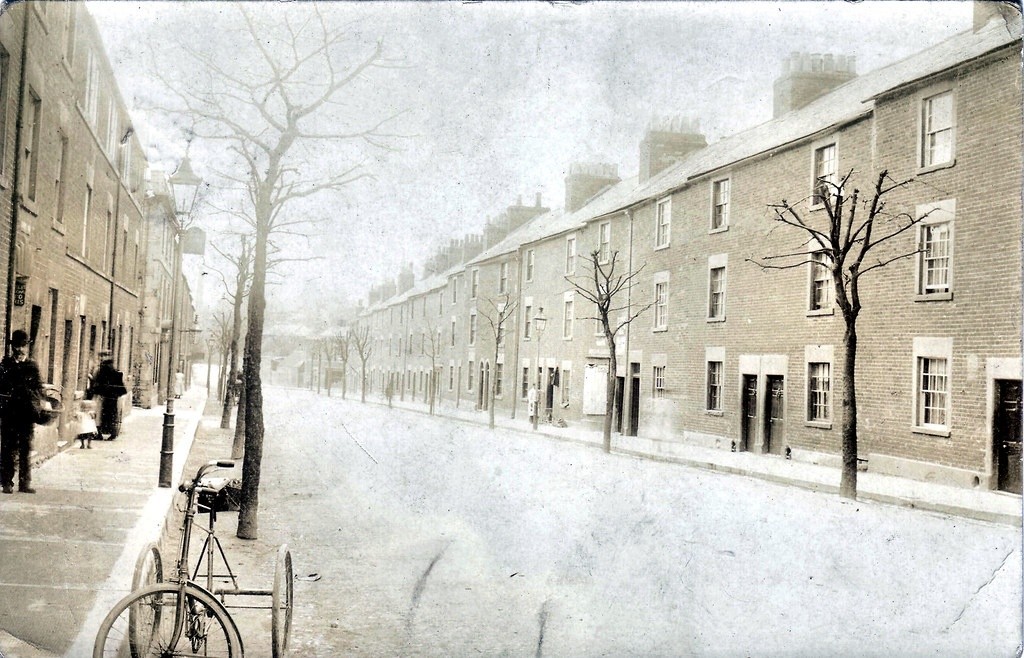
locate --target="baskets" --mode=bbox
[31,382,65,426]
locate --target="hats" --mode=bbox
[98,349,115,364]
[7,330,34,346]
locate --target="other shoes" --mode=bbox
[93,430,103,440]
[87,445,92,449]
[80,445,84,448]
[3,484,13,493]
[19,487,36,493]
[106,435,118,440]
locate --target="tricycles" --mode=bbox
[91,463,295,658]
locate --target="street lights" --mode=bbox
[206,333,216,399]
[157,154,206,490]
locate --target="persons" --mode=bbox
[77,400,98,449]
[237,371,243,381]
[527,383,540,423]
[86,350,127,440]
[0,330,47,494]
[174,369,185,399]
